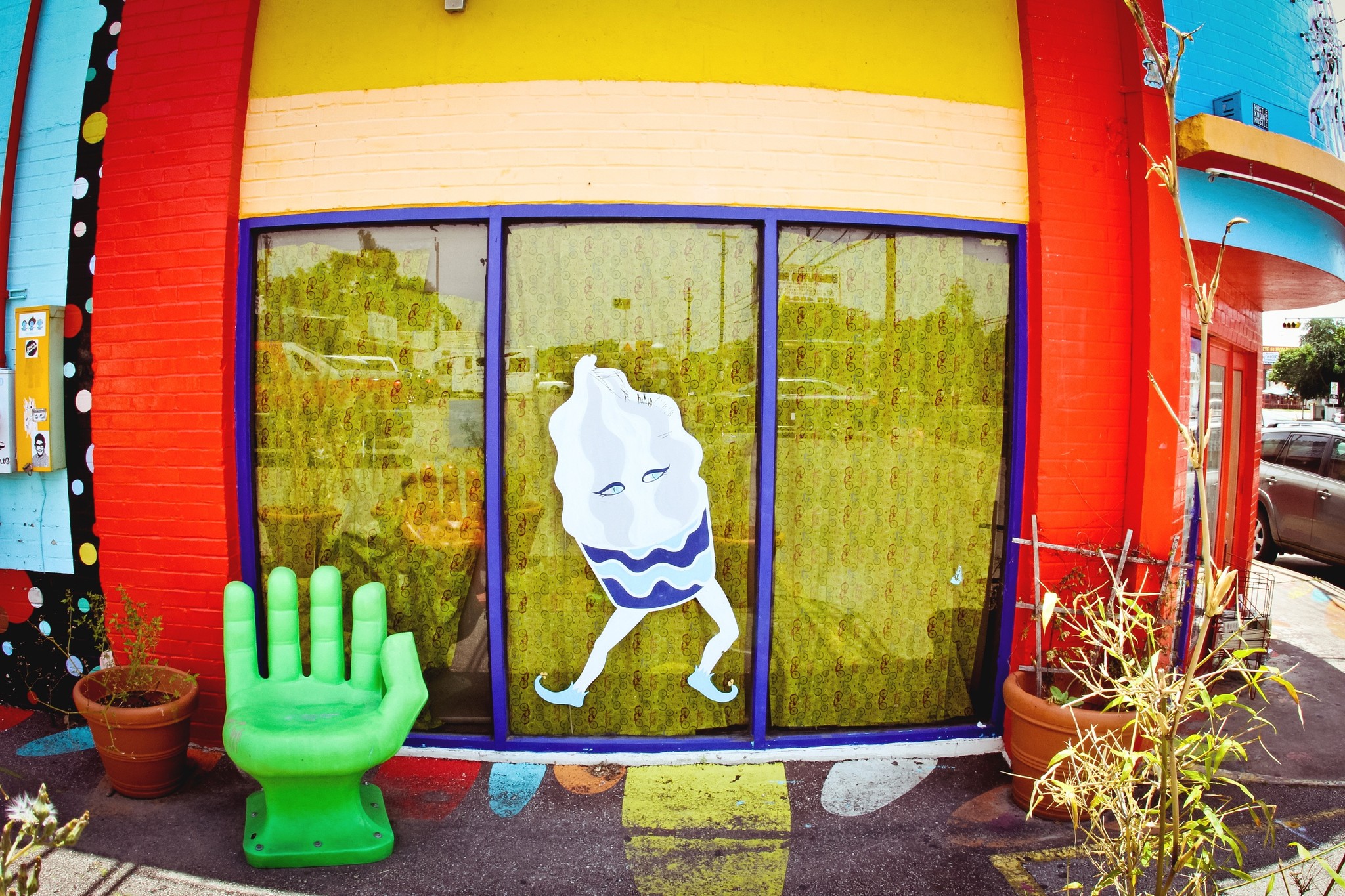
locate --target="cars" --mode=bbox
[1254,421,1345,573]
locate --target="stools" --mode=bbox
[222,566,429,868]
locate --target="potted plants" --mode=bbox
[1001,537,1194,820]
[11,582,199,798]
[258,372,401,579]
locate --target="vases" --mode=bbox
[712,525,784,607]
[476,505,542,571]
